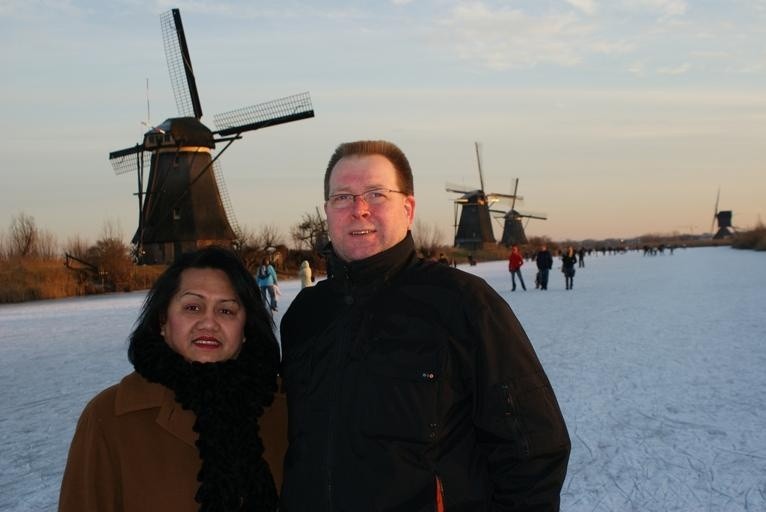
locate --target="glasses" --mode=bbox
[329,186,402,209]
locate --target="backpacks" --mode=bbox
[257,265,269,279]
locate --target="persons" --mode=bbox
[255,256,279,311]
[562,246,577,290]
[59,243,289,512]
[298,260,312,288]
[280,140,571,512]
[525,242,676,268]
[536,244,552,290]
[508,245,527,292]
[424,252,476,269]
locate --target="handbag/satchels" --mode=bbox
[311,276,315,282]
